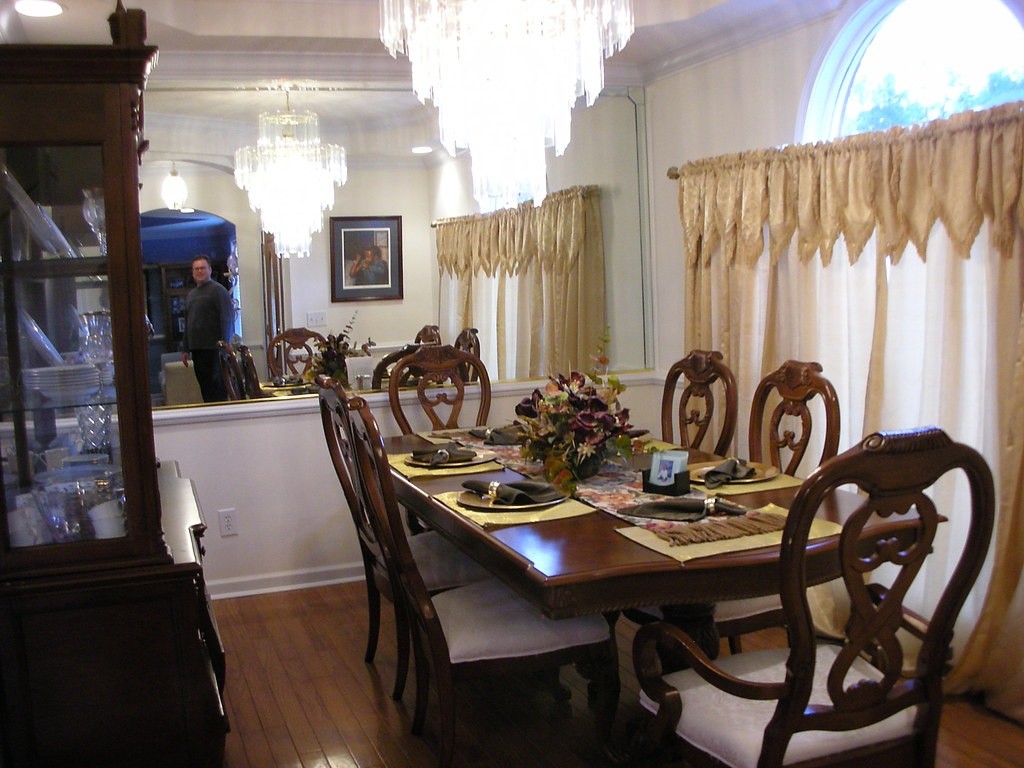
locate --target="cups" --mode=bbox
[355,374,372,391]
[7,446,130,548]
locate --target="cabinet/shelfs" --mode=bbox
[0,7,234,767]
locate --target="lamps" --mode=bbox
[228,82,346,259]
[381,0,637,212]
[163,161,188,211]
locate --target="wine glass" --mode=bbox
[79,311,115,402]
[82,185,107,256]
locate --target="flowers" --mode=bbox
[305,311,377,387]
[484,305,685,491]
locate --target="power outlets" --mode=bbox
[219,507,241,538]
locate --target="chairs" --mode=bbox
[323,340,996,767]
[216,325,481,402]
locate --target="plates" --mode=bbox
[404,450,496,467]
[689,460,780,482]
[17,306,113,400]
[456,490,571,509]
[0,160,77,259]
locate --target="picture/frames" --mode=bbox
[329,214,405,302]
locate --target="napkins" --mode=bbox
[411,443,476,464]
[703,456,755,489]
[614,492,745,521]
[471,426,526,445]
[461,479,566,502]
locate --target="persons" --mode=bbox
[182,255,235,403]
[349,246,388,285]
[657,464,668,482]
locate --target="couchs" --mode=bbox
[158,351,206,408]
